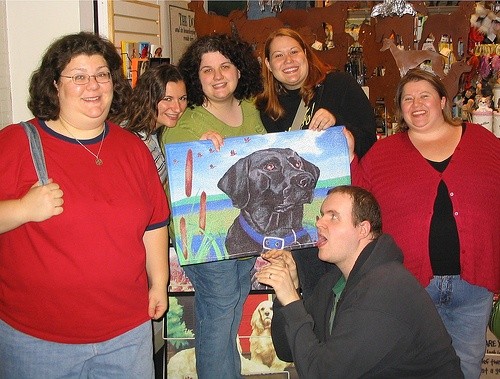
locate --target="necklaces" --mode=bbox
[56,116,106,165]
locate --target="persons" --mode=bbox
[254,28,376,301]
[254,185,464,379]
[342,69,500,379]
[109,64,187,191]
[156,33,267,379]
[0,31,171,379]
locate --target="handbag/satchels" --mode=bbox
[489,300,500,340]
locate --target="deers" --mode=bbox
[380,29,446,80]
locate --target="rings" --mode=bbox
[268,273,273,280]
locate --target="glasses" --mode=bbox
[60,72,113,85]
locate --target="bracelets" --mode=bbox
[296,287,303,295]
